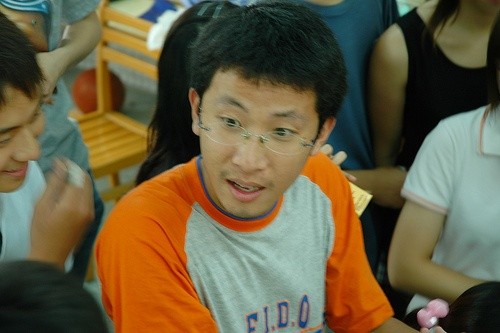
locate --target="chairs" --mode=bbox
[69,0,164,281]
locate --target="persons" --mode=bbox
[367,0,500,255]
[0,11,97,274]
[280,0,404,263]
[384,8,500,333]
[0,1,108,287]
[92,1,450,332]
[134,1,246,192]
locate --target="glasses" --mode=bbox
[197,105,320,155]
